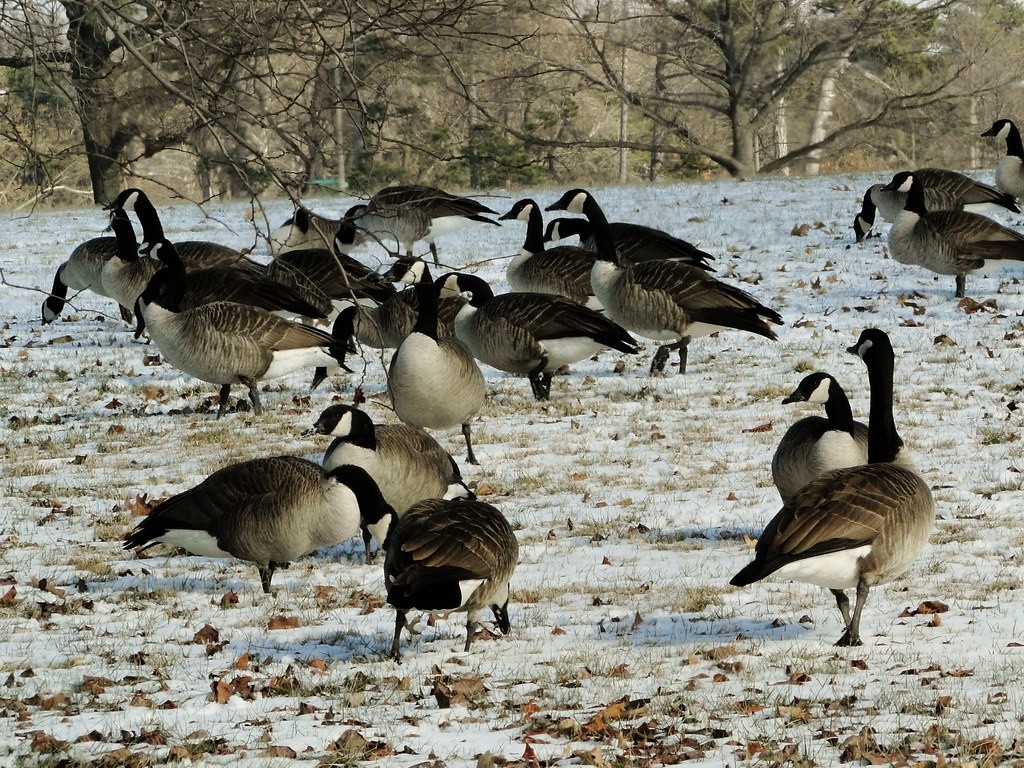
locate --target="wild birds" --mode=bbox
[852,118,1024,303]
[39,126,787,656]
[729,327,935,647]
